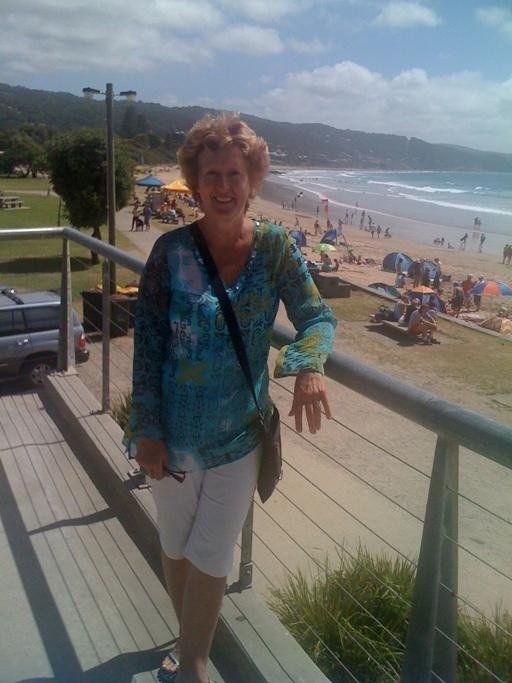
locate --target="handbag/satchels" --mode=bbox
[255,405,280,502]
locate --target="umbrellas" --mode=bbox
[135,174,164,186]
[161,178,192,192]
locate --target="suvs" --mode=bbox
[0,284,92,391]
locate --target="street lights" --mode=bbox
[80,83,137,294]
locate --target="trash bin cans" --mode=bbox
[80,290,138,343]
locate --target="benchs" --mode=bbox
[0,195,23,209]
[368,312,434,344]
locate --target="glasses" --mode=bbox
[161,466,187,483]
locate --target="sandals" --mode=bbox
[161,639,213,683]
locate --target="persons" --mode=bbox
[129,187,511,345]
[121,111,339,683]
